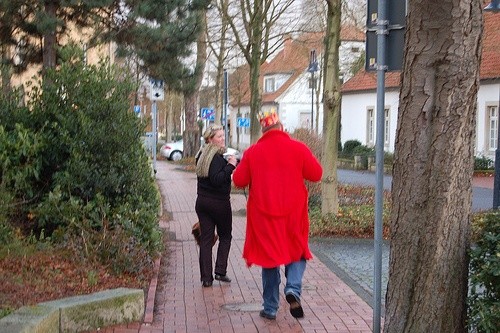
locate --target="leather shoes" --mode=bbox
[215,274,231,282]
[286,292,303,318]
[203,281,212,287]
[260,310,276,319]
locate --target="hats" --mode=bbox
[256,107,280,127]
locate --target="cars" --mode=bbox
[160,137,241,161]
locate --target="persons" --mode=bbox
[232,106,323,320]
[194,124,237,287]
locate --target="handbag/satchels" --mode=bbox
[193,222,218,247]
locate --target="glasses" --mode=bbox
[206,125,223,138]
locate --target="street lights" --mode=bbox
[307,49,319,128]
